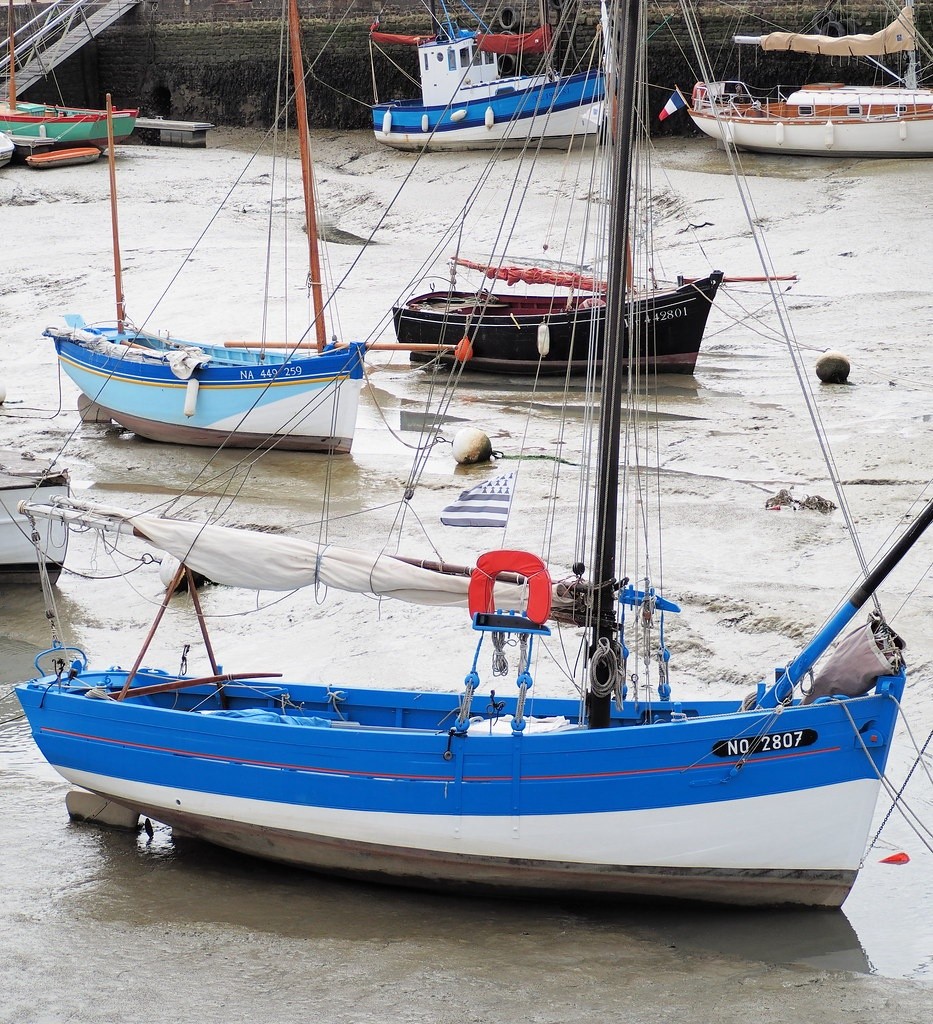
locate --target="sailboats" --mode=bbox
[0,0,140,155]
[389,0,802,375]
[37,0,475,458]
[7,0,932,911]
[363,0,622,152]
[674,0,933,159]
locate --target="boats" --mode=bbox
[0,127,16,170]
[4,125,54,163]
[23,146,102,170]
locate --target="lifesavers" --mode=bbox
[467,549,553,625]
[578,298,606,309]
[812,10,857,37]
[497,5,520,76]
[692,82,705,106]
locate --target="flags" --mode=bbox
[440,473,514,528]
[659,90,685,121]
